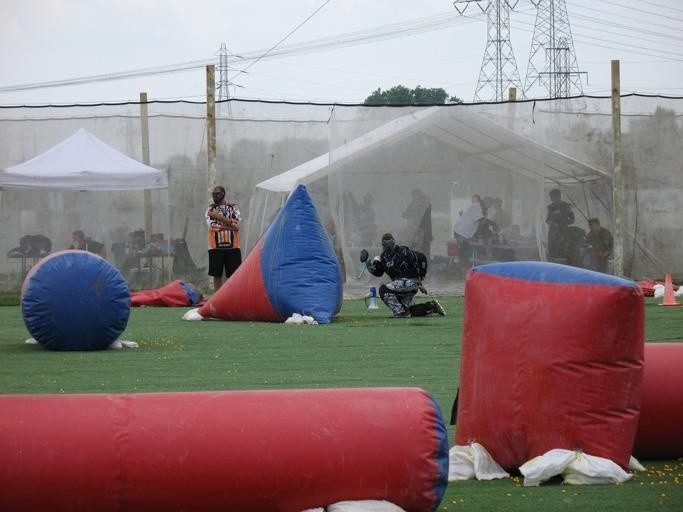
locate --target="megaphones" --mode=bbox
[365,287,378,309]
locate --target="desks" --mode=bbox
[446,234,566,270]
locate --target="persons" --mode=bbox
[454,195,520,267]
[336,189,433,283]
[360,233,447,318]
[204,186,241,291]
[73,231,108,258]
[546,190,575,262]
[586,218,613,271]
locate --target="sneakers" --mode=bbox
[430,299,447,316]
[388,313,411,318]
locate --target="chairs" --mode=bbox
[6,230,206,289]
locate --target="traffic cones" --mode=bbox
[657,273,680,306]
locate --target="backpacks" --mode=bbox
[415,250,427,281]
